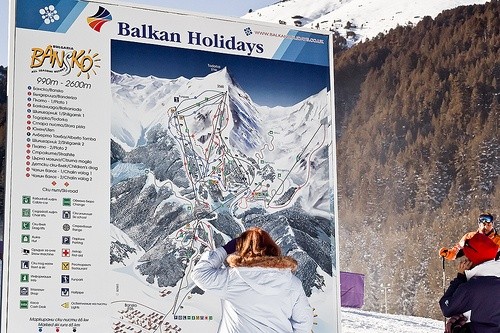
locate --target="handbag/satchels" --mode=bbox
[444,314,471,333]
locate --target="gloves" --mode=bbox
[439,247,449,258]
[221,237,238,254]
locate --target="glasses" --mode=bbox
[478,216,493,224]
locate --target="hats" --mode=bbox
[462,233,499,266]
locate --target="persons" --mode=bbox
[191,228,313,333]
[439,234,500,333]
[439,213,500,261]
